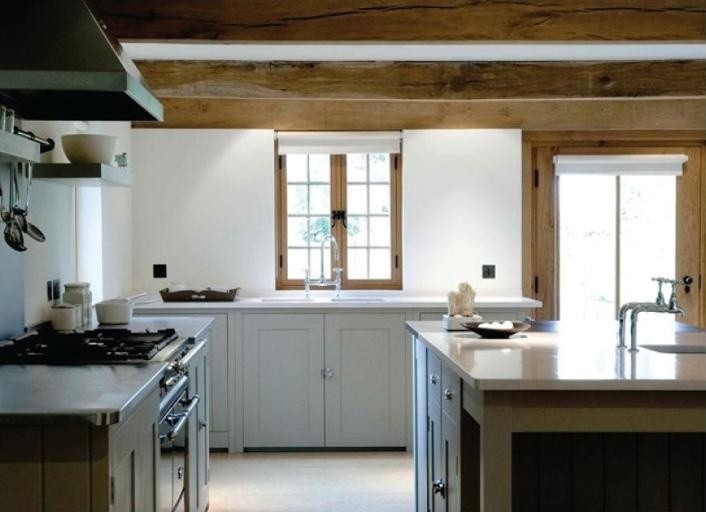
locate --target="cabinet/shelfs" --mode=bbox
[239,309,411,449]
[412,309,525,321]
[136,308,231,438]
[0,384,164,512]
[175,334,211,511]
[414,335,706,511]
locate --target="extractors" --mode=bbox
[0,0,165,121]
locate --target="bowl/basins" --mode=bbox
[61,134,117,164]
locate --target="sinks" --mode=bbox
[262,297,311,304]
[331,297,385,302]
[640,344,706,354]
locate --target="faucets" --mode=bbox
[321,235,339,280]
[616,277,669,348]
[628,279,685,352]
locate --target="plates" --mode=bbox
[458,322,532,339]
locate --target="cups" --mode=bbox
[49,306,76,335]
[73,303,82,329]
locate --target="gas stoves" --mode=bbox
[0,324,208,401]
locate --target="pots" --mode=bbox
[94,291,147,325]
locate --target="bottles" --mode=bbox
[62,281,93,328]
[0,105,15,135]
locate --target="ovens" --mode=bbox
[151,337,200,512]
